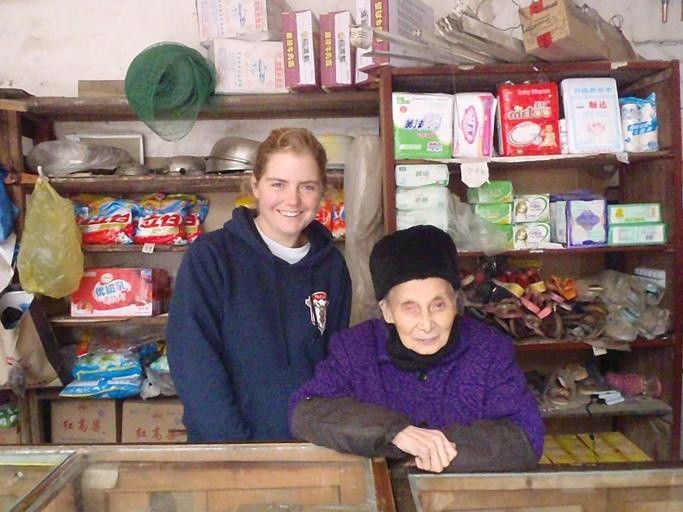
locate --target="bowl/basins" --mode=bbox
[112,136,263,177]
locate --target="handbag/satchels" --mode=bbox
[16,176,87,302]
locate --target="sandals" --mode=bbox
[461,256,675,342]
[532,362,665,409]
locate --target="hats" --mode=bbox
[366,224,459,301]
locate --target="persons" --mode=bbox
[286,225,546,476]
[163,127,352,440]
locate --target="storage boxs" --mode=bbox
[50,398,121,444]
[120,400,188,444]
[195,0,433,96]
[519,0,635,61]
[70,267,169,317]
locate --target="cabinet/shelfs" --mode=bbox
[391,466,683,511]
[371,59,681,471]
[0,85,380,447]
[0,446,397,512]
